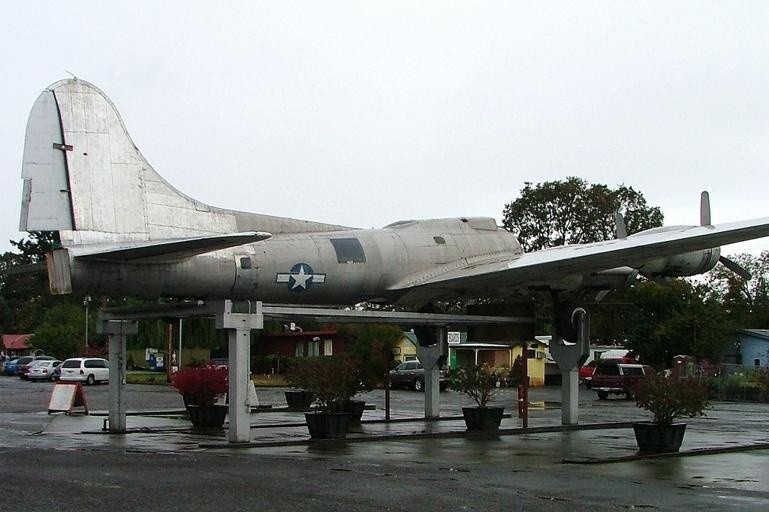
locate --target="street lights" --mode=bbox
[83,297,91,345]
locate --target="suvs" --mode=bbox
[384,361,449,393]
[580,357,658,401]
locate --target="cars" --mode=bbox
[1,355,64,382]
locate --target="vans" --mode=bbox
[58,358,110,385]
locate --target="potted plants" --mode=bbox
[170,363,229,431]
[283,355,367,437]
[440,363,513,431]
[621,362,713,455]
